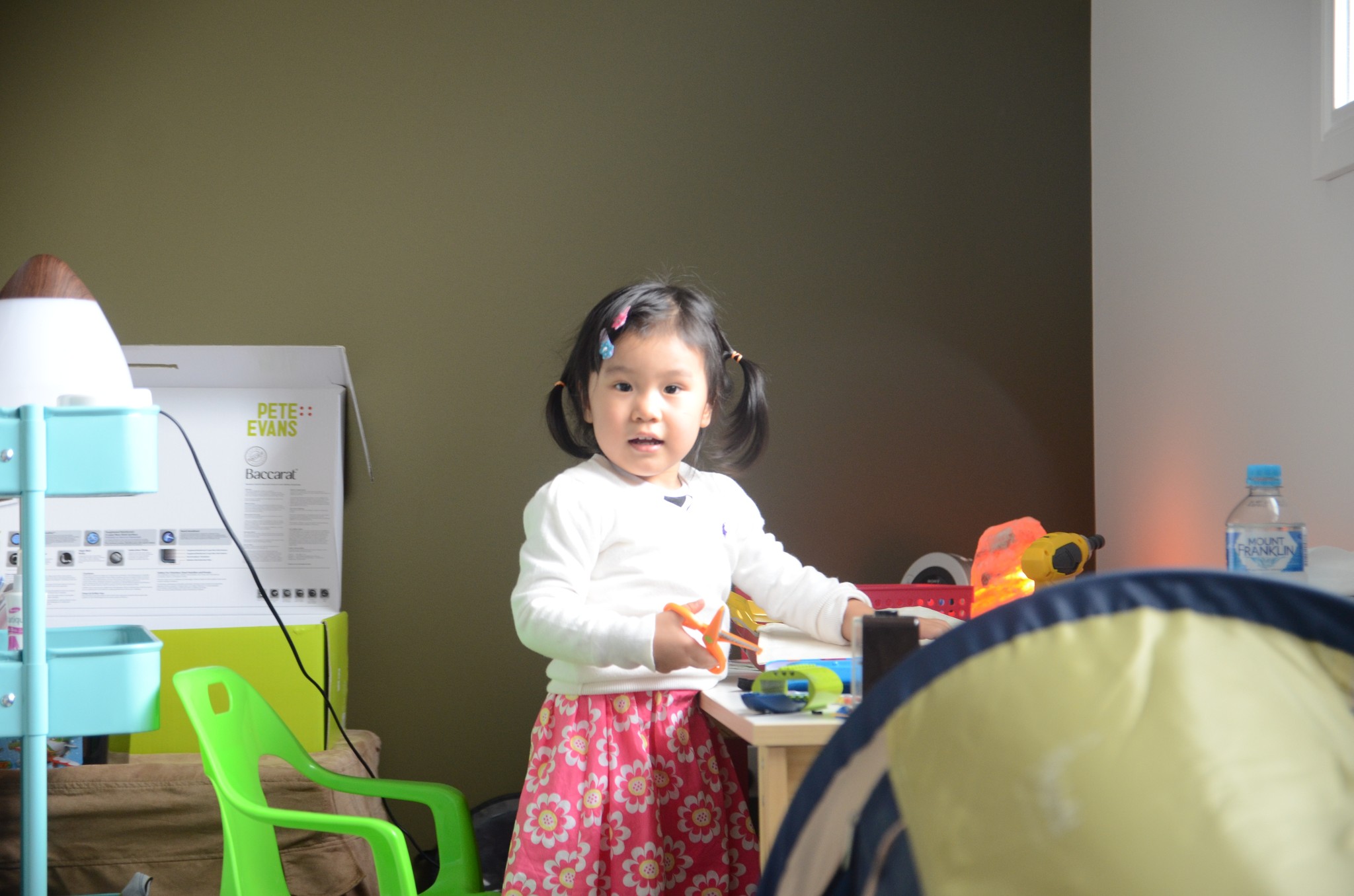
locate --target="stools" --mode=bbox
[0,728,391,896]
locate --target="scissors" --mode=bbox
[664,602,763,674]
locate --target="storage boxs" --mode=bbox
[0,346,374,771]
[734,584,974,673]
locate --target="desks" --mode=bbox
[698,670,864,880]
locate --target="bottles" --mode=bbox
[1224,464,1309,587]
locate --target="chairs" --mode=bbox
[171,664,481,896]
[753,566,1354,896]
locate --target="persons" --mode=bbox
[502,281,951,896]
